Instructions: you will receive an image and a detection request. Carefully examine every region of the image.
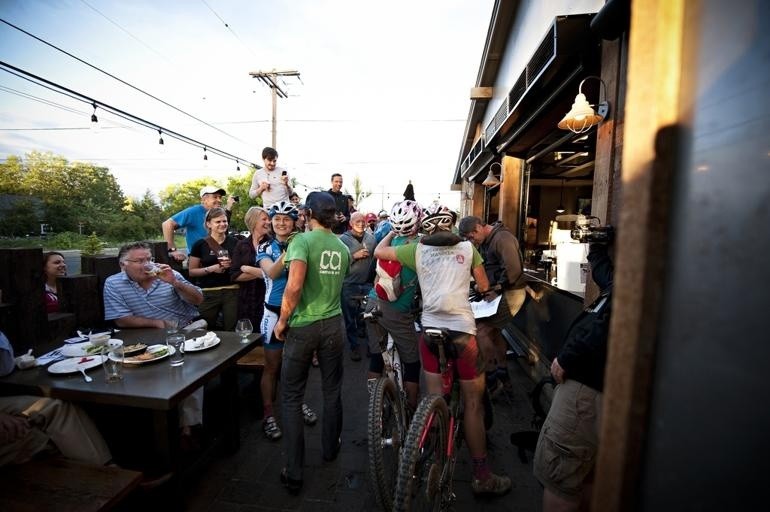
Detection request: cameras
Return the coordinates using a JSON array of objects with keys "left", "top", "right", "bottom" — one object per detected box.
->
[{"left": 233, "top": 196, "right": 240, "bottom": 202}]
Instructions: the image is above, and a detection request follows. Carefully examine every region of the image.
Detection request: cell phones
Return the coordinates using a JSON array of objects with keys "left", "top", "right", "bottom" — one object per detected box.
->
[
  {"left": 64, "top": 336, "right": 89, "bottom": 345},
  {"left": 281, "top": 170, "right": 287, "bottom": 176}
]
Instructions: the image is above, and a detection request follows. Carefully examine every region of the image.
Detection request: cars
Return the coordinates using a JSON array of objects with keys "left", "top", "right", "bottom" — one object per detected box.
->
[{"left": 235, "top": 230, "right": 250, "bottom": 238}]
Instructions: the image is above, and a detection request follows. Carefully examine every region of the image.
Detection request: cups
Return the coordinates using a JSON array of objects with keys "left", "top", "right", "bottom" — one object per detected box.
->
[
  {"left": 141, "top": 261, "right": 167, "bottom": 279},
  {"left": 166, "top": 333, "right": 186, "bottom": 367},
  {"left": 217, "top": 249, "right": 229, "bottom": 267},
  {"left": 101, "top": 344, "right": 124, "bottom": 384}
]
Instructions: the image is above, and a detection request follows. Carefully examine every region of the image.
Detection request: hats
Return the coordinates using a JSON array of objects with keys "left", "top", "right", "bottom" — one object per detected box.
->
[
  {"left": 366, "top": 210, "right": 389, "bottom": 222},
  {"left": 199, "top": 185, "right": 226, "bottom": 198}
]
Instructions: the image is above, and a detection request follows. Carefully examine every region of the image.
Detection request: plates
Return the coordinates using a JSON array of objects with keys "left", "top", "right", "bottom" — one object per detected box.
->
[
  {"left": 183, "top": 336, "right": 222, "bottom": 352},
  {"left": 114, "top": 343, "right": 147, "bottom": 358},
  {"left": 47, "top": 354, "right": 109, "bottom": 374},
  {"left": 62, "top": 338, "right": 123, "bottom": 357},
  {"left": 108, "top": 344, "right": 173, "bottom": 364}
]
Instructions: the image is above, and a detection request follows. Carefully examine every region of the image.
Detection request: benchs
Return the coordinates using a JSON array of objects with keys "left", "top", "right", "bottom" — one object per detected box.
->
[
  {"left": 234, "top": 344, "right": 266, "bottom": 392},
  {"left": 0, "top": 449, "right": 148, "bottom": 512}
]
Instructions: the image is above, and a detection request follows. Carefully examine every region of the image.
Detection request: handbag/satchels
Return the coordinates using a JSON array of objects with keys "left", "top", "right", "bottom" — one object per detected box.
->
[{"left": 375, "top": 258, "right": 403, "bottom": 302}]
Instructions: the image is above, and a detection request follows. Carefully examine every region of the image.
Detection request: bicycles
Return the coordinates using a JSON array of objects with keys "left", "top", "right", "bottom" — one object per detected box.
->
[
  {"left": 352, "top": 294, "right": 424, "bottom": 512},
  {"left": 388, "top": 283, "right": 507, "bottom": 512}
]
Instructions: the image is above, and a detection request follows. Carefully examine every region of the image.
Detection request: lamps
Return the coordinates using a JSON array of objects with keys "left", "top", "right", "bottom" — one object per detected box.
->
[
  {"left": 555, "top": 174, "right": 568, "bottom": 214},
  {"left": 480, "top": 157, "right": 505, "bottom": 192},
  {"left": 556, "top": 73, "right": 611, "bottom": 139}
]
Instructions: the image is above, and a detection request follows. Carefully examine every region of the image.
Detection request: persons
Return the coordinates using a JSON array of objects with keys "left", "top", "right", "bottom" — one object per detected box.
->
[
  {"left": 44, "top": 251, "right": 70, "bottom": 313},
  {"left": 0, "top": 331, "right": 182, "bottom": 490},
  {"left": 101, "top": 146, "right": 617, "bottom": 511}
]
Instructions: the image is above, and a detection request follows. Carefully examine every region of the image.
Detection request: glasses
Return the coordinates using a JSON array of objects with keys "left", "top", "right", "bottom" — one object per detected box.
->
[
  {"left": 467, "top": 230, "right": 476, "bottom": 241},
  {"left": 124, "top": 257, "right": 156, "bottom": 264}
]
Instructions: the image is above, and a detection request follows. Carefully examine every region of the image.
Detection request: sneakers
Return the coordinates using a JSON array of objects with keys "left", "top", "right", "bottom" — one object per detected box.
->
[
  {"left": 472, "top": 472, "right": 511, "bottom": 497},
  {"left": 301, "top": 403, "right": 317, "bottom": 425},
  {"left": 262, "top": 416, "right": 283, "bottom": 440},
  {"left": 351, "top": 346, "right": 362, "bottom": 361}
]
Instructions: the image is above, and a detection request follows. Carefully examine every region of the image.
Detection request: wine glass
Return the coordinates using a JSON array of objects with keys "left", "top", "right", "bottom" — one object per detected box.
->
[{"left": 235, "top": 319, "right": 253, "bottom": 344}]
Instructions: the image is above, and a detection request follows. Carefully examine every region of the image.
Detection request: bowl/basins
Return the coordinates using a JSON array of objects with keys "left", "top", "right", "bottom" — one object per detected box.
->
[{"left": 87, "top": 334, "right": 112, "bottom": 346}]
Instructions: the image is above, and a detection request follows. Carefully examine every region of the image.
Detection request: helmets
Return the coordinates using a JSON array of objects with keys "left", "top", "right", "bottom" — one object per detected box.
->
[
  {"left": 268, "top": 200, "right": 299, "bottom": 221},
  {"left": 421, "top": 204, "right": 453, "bottom": 231},
  {"left": 390, "top": 200, "right": 421, "bottom": 236}
]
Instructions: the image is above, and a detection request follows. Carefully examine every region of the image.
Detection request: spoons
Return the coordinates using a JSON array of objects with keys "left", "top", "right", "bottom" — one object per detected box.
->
[{"left": 76, "top": 365, "right": 93, "bottom": 383}]
